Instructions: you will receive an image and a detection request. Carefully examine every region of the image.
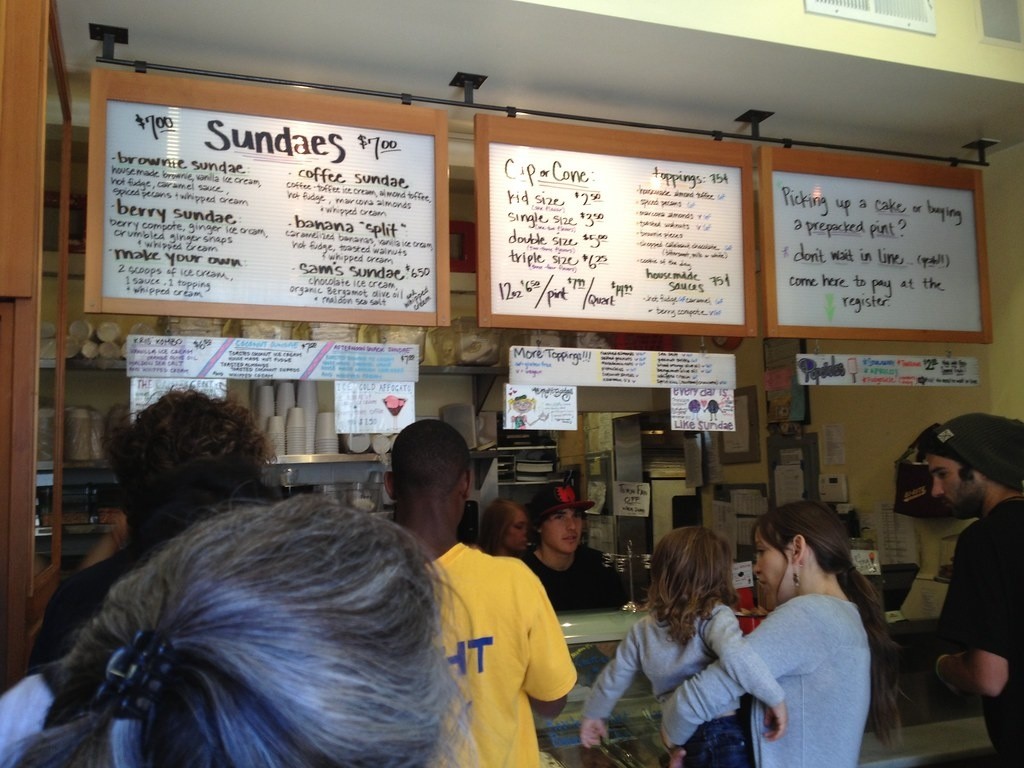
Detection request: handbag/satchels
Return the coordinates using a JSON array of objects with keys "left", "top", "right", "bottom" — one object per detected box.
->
[{"left": 893, "top": 423, "right": 946, "bottom": 519}]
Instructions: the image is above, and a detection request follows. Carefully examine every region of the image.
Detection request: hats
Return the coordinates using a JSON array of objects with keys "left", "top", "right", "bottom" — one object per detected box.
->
[
  {"left": 527, "top": 483, "right": 596, "bottom": 516},
  {"left": 934, "top": 412, "right": 1024, "bottom": 491}
]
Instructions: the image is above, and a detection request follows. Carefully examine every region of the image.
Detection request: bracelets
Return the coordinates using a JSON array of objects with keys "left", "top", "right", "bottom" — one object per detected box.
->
[{"left": 936, "top": 655, "right": 952, "bottom": 679}]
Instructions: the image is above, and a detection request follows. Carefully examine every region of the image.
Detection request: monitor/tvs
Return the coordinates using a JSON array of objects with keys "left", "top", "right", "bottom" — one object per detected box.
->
[{"left": 864, "top": 562, "right": 918, "bottom": 610}]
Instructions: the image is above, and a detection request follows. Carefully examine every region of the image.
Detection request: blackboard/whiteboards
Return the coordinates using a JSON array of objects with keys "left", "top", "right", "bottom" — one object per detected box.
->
[
  {"left": 472, "top": 114, "right": 761, "bottom": 336},
  {"left": 85, "top": 69, "right": 452, "bottom": 326},
  {"left": 757, "top": 143, "right": 995, "bottom": 346}
]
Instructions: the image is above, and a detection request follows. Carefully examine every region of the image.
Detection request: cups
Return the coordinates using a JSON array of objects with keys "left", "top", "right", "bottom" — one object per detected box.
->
[
  {"left": 41, "top": 320, "right": 155, "bottom": 360},
  {"left": 251, "top": 381, "right": 397, "bottom": 456}
]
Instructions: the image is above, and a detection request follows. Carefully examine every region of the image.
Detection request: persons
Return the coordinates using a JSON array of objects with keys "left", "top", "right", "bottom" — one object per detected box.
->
[
  {"left": 0, "top": 388, "right": 465, "bottom": 768},
  {"left": 579, "top": 525, "right": 789, "bottom": 768},
  {"left": 384, "top": 418, "right": 577, "bottom": 768},
  {"left": 660, "top": 500, "right": 903, "bottom": 768},
  {"left": 521, "top": 482, "right": 629, "bottom": 682},
  {"left": 479, "top": 497, "right": 529, "bottom": 558},
  {"left": 927, "top": 412, "right": 1024, "bottom": 768}
]
